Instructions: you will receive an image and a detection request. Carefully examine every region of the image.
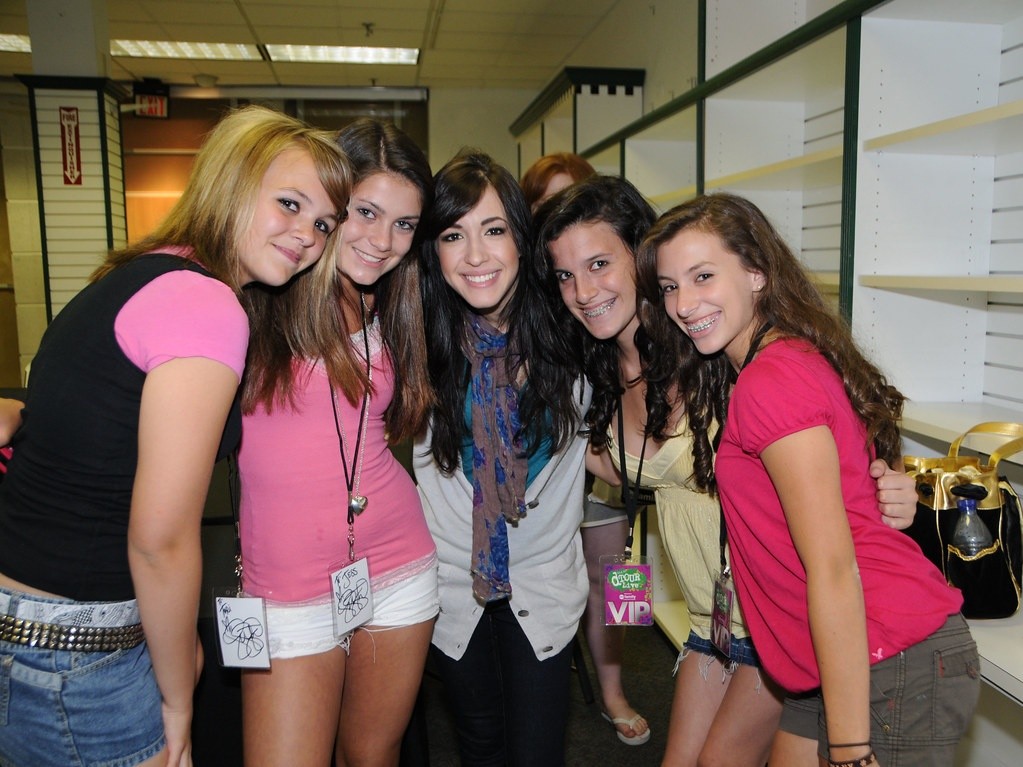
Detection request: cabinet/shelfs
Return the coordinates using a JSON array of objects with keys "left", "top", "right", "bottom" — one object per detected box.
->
[{"left": 508, "top": 0, "right": 1022, "bottom": 708}]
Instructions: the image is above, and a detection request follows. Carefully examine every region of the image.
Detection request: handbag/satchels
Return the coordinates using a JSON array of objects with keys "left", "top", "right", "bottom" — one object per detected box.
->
[{"left": 903, "top": 422, "right": 1023, "bottom": 620}]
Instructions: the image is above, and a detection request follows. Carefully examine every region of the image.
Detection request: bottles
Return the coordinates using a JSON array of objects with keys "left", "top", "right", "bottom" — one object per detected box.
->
[{"left": 954, "top": 499, "right": 993, "bottom": 556}]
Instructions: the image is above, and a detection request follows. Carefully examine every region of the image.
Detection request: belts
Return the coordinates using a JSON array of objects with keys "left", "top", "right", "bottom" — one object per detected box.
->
[{"left": 0, "top": 615, "right": 145, "bottom": 652}]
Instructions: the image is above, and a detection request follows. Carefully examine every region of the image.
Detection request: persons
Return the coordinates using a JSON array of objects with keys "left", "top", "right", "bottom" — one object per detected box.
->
[
  {"left": 523, "top": 154, "right": 651, "bottom": 745},
  {"left": 527, "top": 176, "right": 784, "bottom": 766},
  {"left": 0, "top": 118, "right": 440, "bottom": 766},
  {"left": 634, "top": 194, "right": 980, "bottom": 767},
  {"left": 413, "top": 156, "right": 595, "bottom": 766},
  {"left": 0, "top": 104, "right": 352, "bottom": 766}
]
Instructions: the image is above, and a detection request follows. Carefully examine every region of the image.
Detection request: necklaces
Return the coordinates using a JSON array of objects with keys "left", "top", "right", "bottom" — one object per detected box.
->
[
  {"left": 333, "top": 290, "right": 373, "bottom": 516},
  {"left": 626, "top": 376, "right": 643, "bottom": 388}
]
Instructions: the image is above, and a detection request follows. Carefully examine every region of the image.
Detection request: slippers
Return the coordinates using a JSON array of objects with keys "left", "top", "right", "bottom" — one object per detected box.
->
[{"left": 601, "top": 708, "right": 650, "bottom": 745}]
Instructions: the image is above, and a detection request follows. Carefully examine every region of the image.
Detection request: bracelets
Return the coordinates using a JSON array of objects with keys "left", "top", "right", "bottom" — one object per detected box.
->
[
  {"left": 827, "top": 742, "right": 871, "bottom": 748},
  {"left": 828, "top": 751, "right": 875, "bottom": 767}
]
